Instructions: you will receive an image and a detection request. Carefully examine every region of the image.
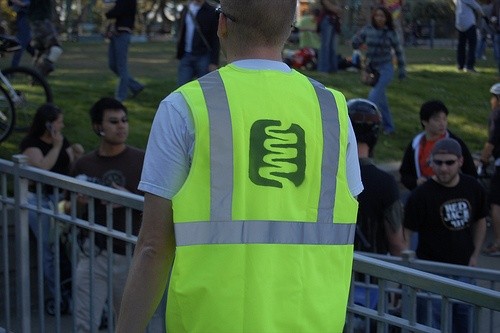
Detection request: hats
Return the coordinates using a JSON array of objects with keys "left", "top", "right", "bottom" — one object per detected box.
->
[{"left": 432, "top": 138, "right": 462, "bottom": 158}]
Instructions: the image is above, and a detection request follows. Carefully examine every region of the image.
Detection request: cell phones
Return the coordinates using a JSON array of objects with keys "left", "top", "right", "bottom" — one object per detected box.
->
[{"left": 46, "top": 122, "right": 56, "bottom": 137}]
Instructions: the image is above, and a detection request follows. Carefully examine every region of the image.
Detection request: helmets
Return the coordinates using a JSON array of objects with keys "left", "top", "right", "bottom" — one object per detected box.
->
[
  {"left": 489, "top": 83, "right": 500, "bottom": 95},
  {"left": 347, "top": 98, "right": 382, "bottom": 138}
]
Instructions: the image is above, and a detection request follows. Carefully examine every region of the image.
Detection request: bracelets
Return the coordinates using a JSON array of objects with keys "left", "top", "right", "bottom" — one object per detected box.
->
[{"left": 478, "top": 159, "right": 487, "bottom": 165}]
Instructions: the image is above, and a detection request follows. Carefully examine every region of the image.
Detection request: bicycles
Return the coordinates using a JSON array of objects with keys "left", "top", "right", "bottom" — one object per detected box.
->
[{"left": 0, "top": 33, "right": 55, "bottom": 144}]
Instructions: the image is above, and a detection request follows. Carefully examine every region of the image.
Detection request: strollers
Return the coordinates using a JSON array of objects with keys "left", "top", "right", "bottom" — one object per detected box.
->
[{"left": 24, "top": 193, "right": 110, "bottom": 331}]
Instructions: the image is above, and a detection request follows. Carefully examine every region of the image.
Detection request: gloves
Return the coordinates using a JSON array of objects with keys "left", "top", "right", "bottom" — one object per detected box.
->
[
  {"left": 398, "top": 69, "right": 405, "bottom": 78},
  {"left": 352, "top": 39, "right": 360, "bottom": 49}
]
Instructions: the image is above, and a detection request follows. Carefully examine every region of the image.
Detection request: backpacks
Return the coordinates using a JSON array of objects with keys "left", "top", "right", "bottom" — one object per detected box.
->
[{"left": 285, "top": 47, "right": 353, "bottom": 71}]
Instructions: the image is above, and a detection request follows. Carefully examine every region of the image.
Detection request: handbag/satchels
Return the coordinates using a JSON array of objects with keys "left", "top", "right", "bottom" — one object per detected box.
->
[
  {"left": 360, "top": 65, "right": 381, "bottom": 86},
  {"left": 335, "top": 15, "right": 342, "bottom": 33},
  {"left": 474, "top": 10, "right": 482, "bottom": 24}
]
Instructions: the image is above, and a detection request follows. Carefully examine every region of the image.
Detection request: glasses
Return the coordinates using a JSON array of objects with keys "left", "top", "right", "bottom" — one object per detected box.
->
[
  {"left": 215, "top": 7, "right": 237, "bottom": 23},
  {"left": 101, "top": 118, "right": 128, "bottom": 125},
  {"left": 433, "top": 160, "right": 457, "bottom": 166}
]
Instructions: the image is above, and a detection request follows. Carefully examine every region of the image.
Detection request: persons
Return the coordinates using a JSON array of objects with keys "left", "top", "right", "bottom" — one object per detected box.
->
[
  {"left": 177, "top": 0, "right": 219, "bottom": 90},
  {"left": 315, "top": 0, "right": 344, "bottom": 75},
  {"left": 402, "top": 137, "right": 488, "bottom": 333},
  {"left": 29, "top": 0, "right": 63, "bottom": 85},
  {"left": 455, "top": 0, "right": 486, "bottom": 74},
  {"left": 352, "top": 6, "right": 407, "bottom": 135},
  {"left": 400, "top": 98, "right": 479, "bottom": 254},
  {"left": 6, "top": 0, "right": 37, "bottom": 79},
  {"left": 342, "top": 97, "right": 405, "bottom": 333},
  {"left": 474, "top": 83, "right": 500, "bottom": 256},
  {"left": 19, "top": 103, "right": 84, "bottom": 317},
  {"left": 72, "top": 95, "right": 146, "bottom": 333},
  {"left": 377, "top": 0, "right": 404, "bottom": 64},
  {"left": 103, "top": 0, "right": 144, "bottom": 102},
  {"left": 475, "top": 0, "right": 500, "bottom": 64},
  {"left": 113, "top": 0, "right": 365, "bottom": 333}
]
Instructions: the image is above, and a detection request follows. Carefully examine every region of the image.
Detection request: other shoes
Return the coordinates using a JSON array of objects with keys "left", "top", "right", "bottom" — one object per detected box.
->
[
  {"left": 457, "top": 52, "right": 500, "bottom": 79},
  {"left": 134, "top": 88, "right": 151, "bottom": 99}
]
